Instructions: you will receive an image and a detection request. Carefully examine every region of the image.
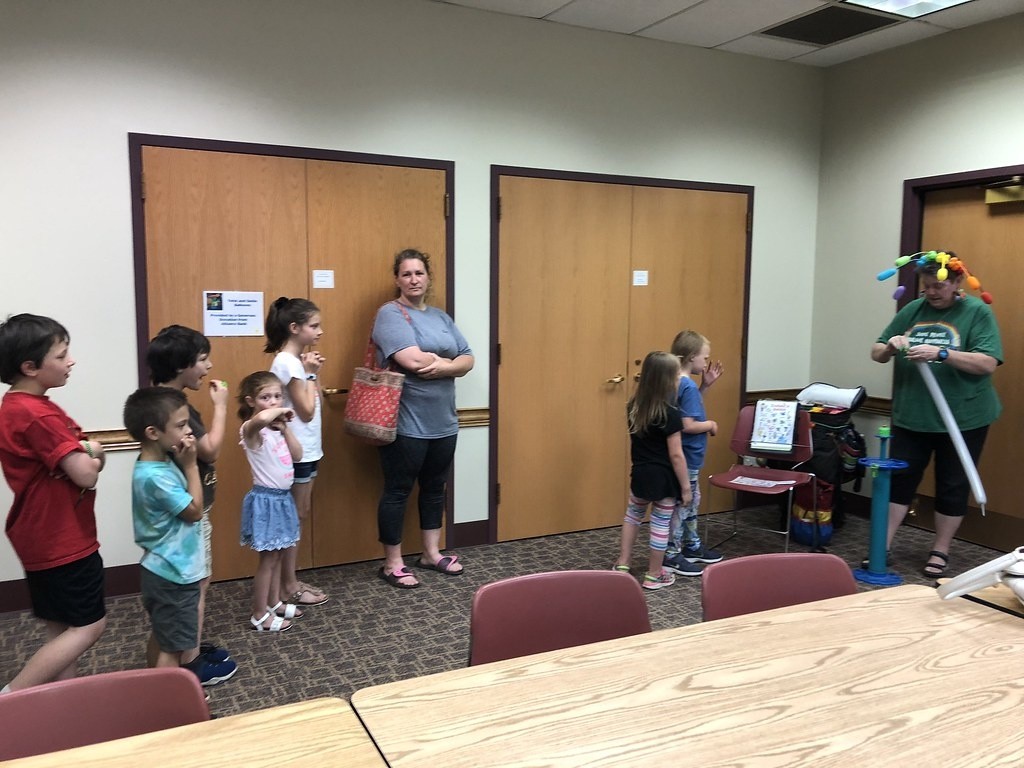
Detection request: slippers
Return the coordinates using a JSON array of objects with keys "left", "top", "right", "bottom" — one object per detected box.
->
[
  {"left": 378, "top": 565, "right": 419, "bottom": 588},
  {"left": 417, "top": 554, "right": 464, "bottom": 575}
]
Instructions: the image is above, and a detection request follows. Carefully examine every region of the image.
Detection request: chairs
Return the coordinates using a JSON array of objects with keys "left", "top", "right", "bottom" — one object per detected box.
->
[
  {"left": 701, "top": 552, "right": 856, "bottom": 622},
  {"left": 0, "top": 666, "right": 210, "bottom": 762},
  {"left": 705, "top": 406, "right": 819, "bottom": 552},
  {"left": 469, "top": 570, "right": 651, "bottom": 668}
]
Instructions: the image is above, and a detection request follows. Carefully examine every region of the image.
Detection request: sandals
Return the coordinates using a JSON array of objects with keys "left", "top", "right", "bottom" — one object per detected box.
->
[
  {"left": 281, "top": 580, "right": 329, "bottom": 606},
  {"left": 859, "top": 549, "right": 893, "bottom": 569},
  {"left": 249, "top": 600, "right": 305, "bottom": 631},
  {"left": 924, "top": 552, "right": 949, "bottom": 578}
]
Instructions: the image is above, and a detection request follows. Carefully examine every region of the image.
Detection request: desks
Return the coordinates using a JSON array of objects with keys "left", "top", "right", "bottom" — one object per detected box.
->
[
  {"left": 352, "top": 584, "right": 1023, "bottom": 768},
  {"left": 938, "top": 577, "right": 1024, "bottom": 619},
  {"left": 0, "top": 697, "right": 389, "bottom": 768}
]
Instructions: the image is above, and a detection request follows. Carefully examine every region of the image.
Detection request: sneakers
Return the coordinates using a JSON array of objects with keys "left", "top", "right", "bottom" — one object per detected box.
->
[
  {"left": 642, "top": 569, "right": 675, "bottom": 590},
  {"left": 612, "top": 558, "right": 631, "bottom": 572},
  {"left": 179, "top": 645, "right": 239, "bottom": 686},
  {"left": 684, "top": 543, "right": 723, "bottom": 564},
  {"left": 662, "top": 553, "right": 703, "bottom": 576}
]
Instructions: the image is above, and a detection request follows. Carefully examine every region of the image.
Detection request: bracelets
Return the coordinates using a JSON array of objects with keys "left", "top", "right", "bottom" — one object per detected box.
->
[{"left": 83, "top": 440, "right": 93, "bottom": 457}]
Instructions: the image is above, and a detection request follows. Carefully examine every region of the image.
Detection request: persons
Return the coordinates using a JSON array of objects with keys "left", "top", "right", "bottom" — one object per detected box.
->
[
  {"left": 264, "top": 296, "right": 329, "bottom": 607},
  {"left": 144, "top": 325, "right": 238, "bottom": 687},
  {"left": 611, "top": 350, "right": 693, "bottom": 589},
  {"left": 859, "top": 250, "right": 1005, "bottom": 578},
  {"left": 0, "top": 313, "right": 106, "bottom": 695},
  {"left": 123, "top": 387, "right": 210, "bottom": 702},
  {"left": 236, "top": 370, "right": 305, "bottom": 632},
  {"left": 372, "top": 249, "right": 475, "bottom": 589},
  {"left": 661, "top": 328, "right": 724, "bottom": 576}
]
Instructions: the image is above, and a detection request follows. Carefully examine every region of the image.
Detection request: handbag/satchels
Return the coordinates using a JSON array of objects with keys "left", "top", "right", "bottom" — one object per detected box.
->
[{"left": 342, "top": 301, "right": 411, "bottom": 446}]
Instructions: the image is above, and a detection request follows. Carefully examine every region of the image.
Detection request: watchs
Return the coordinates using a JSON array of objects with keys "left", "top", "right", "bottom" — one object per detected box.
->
[
  {"left": 937, "top": 346, "right": 949, "bottom": 362},
  {"left": 306, "top": 374, "right": 316, "bottom": 381}
]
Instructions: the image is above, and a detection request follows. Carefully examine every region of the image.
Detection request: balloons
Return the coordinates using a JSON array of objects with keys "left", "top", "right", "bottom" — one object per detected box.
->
[
  {"left": 913, "top": 360, "right": 987, "bottom": 516},
  {"left": 877, "top": 251, "right": 994, "bottom": 304}
]
